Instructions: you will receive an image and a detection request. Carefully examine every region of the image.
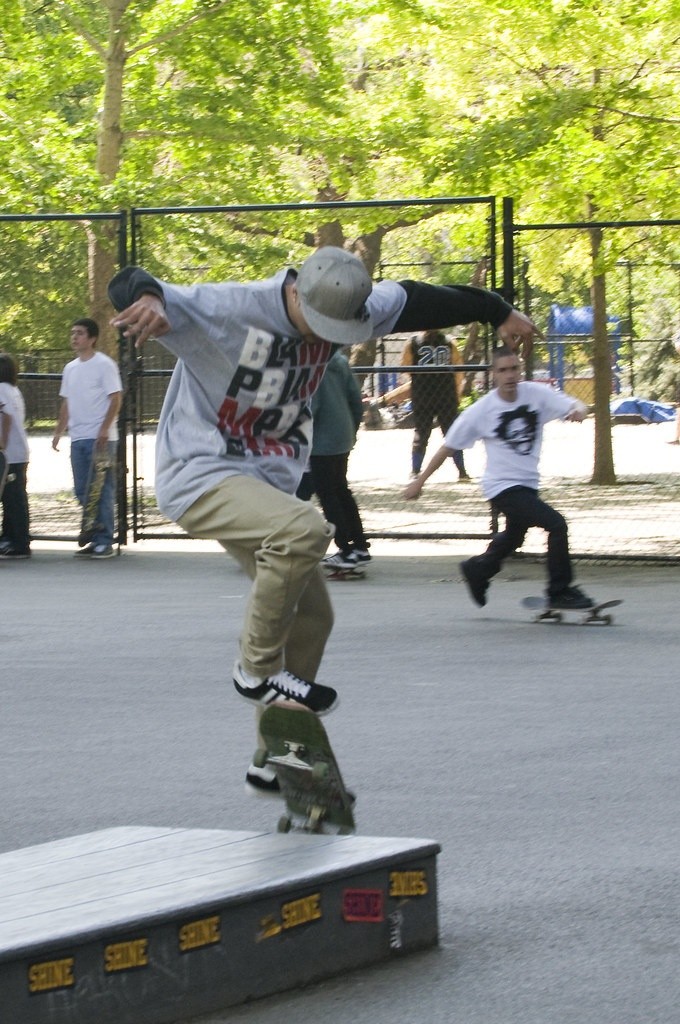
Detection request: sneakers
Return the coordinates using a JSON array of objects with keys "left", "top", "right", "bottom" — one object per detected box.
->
[
  {"left": 90, "top": 545, "right": 113, "bottom": 559},
  {"left": 0, "top": 544, "right": 31, "bottom": 558},
  {"left": 233, "top": 658, "right": 338, "bottom": 717},
  {"left": 73, "top": 544, "right": 95, "bottom": 556},
  {"left": 319, "top": 549, "right": 373, "bottom": 568},
  {"left": 549, "top": 582, "right": 595, "bottom": 608},
  {"left": 244, "top": 763, "right": 358, "bottom": 812},
  {"left": 458, "top": 558, "right": 490, "bottom": 608}
]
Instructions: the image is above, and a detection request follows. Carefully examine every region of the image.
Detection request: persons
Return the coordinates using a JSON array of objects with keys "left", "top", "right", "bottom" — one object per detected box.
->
[
  {"left": 107, "top": 246, "right": 543, "bottom": 805},
  {"left": 53, "top": 319, "right": 122, "bottom": 558},
  {"left": 400, "top": 346, "right": 593, "bottom": 610},
  {"left": 394, "top": 327, "right": 473, "bottom": 484},
  {"left": 293, "top": 352, "right": 372, "bottom": 568},
  {"left": 0, "top": 352, "right": 32, "bottom": 560}
]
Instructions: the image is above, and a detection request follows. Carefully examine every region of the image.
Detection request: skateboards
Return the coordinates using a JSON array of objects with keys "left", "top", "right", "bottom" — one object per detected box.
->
[
  {"left": 76, "top": 438, "right": 112, "bottom": 547},
  {"left": 259, "top": 700, "right": 358, "bottom": 837},
  {"left": 0, "top": 448, "right": 16, "bottom": 501},
  {"left": 321, "top": 563, "right": 370, "bottom": 582},
  {"left": 521, "top": 597, "right": 625, "bottom": 626}
]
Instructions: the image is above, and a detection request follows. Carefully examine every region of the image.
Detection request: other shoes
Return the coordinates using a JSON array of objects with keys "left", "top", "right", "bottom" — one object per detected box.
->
[{"left": 459, "top": 471, "right": 472, "bottom": 480}]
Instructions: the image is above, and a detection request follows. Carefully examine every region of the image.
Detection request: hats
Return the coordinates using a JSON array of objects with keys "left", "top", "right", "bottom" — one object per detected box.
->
[{"left": 296, "top": 246, "right": 373, "bottom": 344}]
